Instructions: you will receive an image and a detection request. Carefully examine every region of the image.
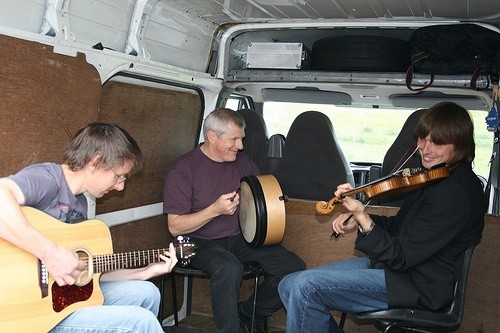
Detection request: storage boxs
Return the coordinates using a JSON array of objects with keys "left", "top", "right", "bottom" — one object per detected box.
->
[{"left": 246, "top": 41, "right": 310, "bottom": 71}]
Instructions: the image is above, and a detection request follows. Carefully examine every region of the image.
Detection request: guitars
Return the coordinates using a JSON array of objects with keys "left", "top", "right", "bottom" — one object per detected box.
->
[{"left": 0, "top": 205, "right": 198, "bottom": 333}]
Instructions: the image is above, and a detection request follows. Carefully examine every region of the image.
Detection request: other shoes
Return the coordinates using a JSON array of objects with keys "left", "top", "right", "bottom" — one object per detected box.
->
[{"left": 236, "top": 302, "right": 274, "bottom": 333}]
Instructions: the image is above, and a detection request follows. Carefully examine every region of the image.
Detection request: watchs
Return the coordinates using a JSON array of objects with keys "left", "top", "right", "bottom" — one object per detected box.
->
[{"left": 358, "top": 220, "right": 375, "bottom": 236}]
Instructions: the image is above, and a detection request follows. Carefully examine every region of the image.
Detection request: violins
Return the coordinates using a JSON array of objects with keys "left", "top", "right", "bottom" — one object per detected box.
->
[{"left": 315, "top": 162, "right": 452, "bottom": 216}]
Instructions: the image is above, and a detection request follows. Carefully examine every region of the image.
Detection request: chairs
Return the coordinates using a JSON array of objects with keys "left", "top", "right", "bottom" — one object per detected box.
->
[
  {"left": 159, "top": 260, "right": 266, "bottom": 333},
  {"left": 340, "top": 238, "right": 482, "bottom": 333},
  {"left": 238, "top": 108, "right": 429, "bottom": 206}
]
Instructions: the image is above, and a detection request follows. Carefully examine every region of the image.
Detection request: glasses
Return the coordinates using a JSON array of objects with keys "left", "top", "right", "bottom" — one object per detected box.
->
[{"left": 101, "top": 160, "right": 128, "bottom": 183}]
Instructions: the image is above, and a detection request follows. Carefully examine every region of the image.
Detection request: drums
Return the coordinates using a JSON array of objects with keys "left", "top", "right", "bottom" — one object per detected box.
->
[{"left": 237, "top": 174, "right": 286, "bottom": 247}]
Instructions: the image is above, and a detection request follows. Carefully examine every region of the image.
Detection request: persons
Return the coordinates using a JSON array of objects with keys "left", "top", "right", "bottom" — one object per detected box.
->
[
  {"left": 0, "top": 123, "right": 178, "bottom": 333},
  {"left": 277, "top": 102, "right": 486, "bottom": 333},
  {"left": 163, "top": 108, "right": 306, "bottom": 333}
]
